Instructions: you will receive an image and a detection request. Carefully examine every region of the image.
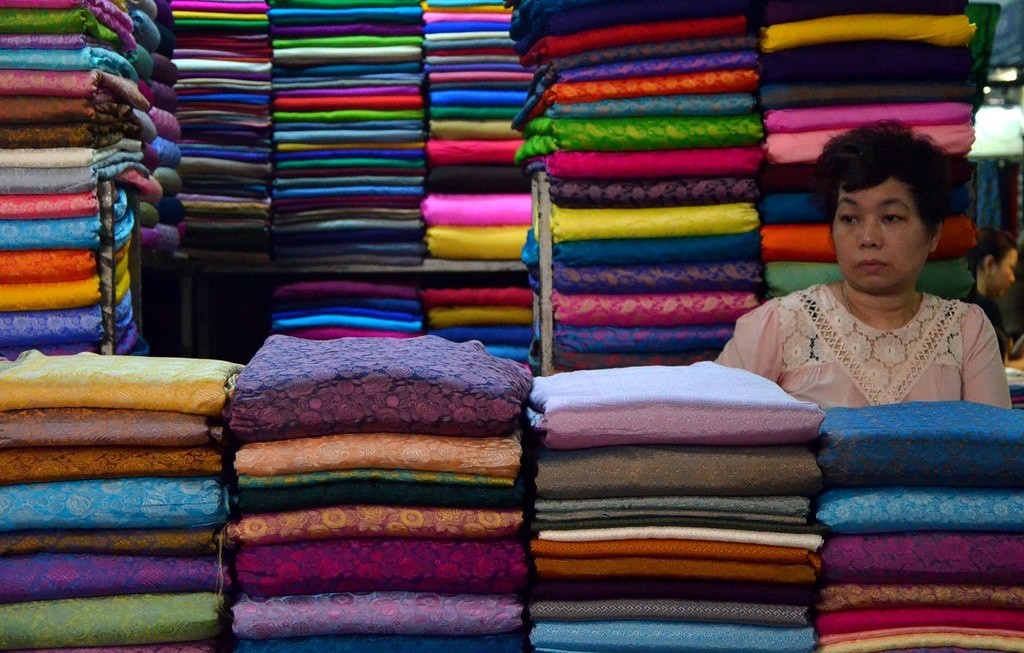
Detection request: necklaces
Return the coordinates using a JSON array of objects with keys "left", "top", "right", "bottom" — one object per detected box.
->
[{"left": 842, "top": 287, "right": 915, "bottom": 317}]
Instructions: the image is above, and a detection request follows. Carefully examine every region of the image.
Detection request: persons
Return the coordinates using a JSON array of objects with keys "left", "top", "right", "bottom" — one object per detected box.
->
[
  {"left": 715, "top": 123, "right": 1011, "bottom": 413},
  {"left": 960, "top": 226, "right": 1018, "bottom": 364}
]
